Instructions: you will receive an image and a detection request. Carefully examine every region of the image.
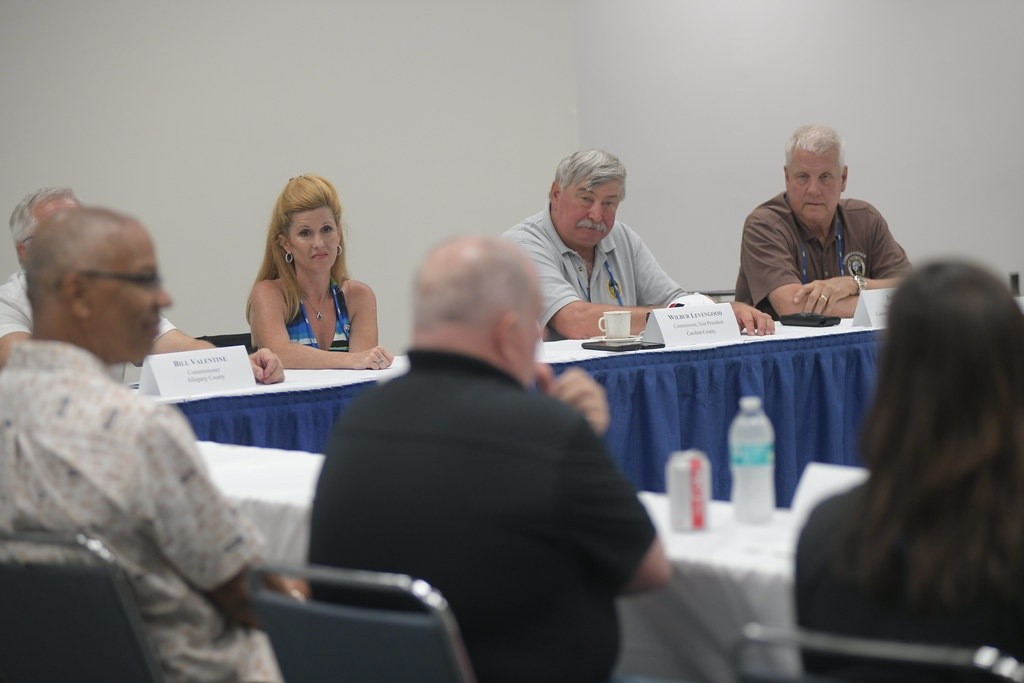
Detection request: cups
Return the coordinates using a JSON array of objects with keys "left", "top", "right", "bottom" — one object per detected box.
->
[{"left": 598, "top": 312, "right": 631, "bottom": 337}]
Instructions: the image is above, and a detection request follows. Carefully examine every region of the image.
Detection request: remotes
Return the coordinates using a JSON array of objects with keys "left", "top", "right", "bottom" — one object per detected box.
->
[{"left": 780, "top": 311, "right": 841, "bottom": 326}]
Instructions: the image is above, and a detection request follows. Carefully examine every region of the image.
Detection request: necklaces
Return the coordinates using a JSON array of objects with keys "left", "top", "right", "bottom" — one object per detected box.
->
[{"left": 303, "top": 292, "right": 330, "bottom": 322}]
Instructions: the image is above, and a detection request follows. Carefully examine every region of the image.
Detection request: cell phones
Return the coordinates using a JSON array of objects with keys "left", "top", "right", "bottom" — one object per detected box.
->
[{"left": 582, "top": 340, "right": 642, "bottom": 352}]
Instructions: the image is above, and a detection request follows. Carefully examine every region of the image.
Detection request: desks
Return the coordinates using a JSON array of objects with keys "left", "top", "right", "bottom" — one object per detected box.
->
[
  {"left": 156, "top": 318, "right": 889, "bottom": 513},
  {"left": 201, "top": 439, "right": 804, "bottom": 683}
]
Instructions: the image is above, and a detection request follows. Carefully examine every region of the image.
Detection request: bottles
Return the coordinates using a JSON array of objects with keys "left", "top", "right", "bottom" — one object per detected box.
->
[{"left": 730, "top": 396, "right": 773, "bottom": 521}]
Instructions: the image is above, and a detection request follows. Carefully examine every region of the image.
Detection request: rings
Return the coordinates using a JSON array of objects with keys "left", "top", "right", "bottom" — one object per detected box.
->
[
  {"left": 378, "top": 359, "right": 384, "bottom": 364},
  {"left": 821, "top": 295, "right": 828, "bottom": 301}
]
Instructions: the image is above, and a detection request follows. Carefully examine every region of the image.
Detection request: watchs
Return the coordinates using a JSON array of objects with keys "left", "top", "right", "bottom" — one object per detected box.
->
[{"left": 853, "top": 275, "right": 867, "bottom": 295}]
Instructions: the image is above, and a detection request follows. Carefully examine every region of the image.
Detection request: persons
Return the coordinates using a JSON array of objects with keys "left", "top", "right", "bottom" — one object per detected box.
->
[
  {"left": 0, "top": 187, "right": 285, "bottom": 385},
  {"left": 243, "top": 176, "right": 394, "bottom": 372},
  {"left": 499, "top": 149, "right": 775, "bottom": 336},
  {"left": 789, "top": 260, "right": 1024, "bottom": 683},
  {"left": 305, "top": 232, "right": 673, "bottom": 683},
  {"left": 0, "top": 207, "right": 308, "bottom": 683},
  {"left": 734, "top": 124, "right": 912, "bottom": 318}
]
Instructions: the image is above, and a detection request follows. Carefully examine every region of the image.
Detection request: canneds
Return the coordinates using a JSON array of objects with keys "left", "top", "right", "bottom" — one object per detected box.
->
[{"left": 665, "top": 448, "right": 713, "bottom": 532}]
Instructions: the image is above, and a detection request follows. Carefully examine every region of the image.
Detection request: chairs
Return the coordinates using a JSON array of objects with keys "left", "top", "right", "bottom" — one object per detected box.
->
[
  {"left": 0, "top": 528, "right": 166, "bottom": 683},
  {"left": 244, "top": 556, "right": 478, "bottom": 683},
  {"left": 730, "top": 621, "right": 1024, "bottom": 683}
]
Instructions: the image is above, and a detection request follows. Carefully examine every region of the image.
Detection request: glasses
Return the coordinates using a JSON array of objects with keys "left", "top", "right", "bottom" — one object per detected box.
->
[{"left": 80, "top": 265, "right": 159, "bottom": 290}]
